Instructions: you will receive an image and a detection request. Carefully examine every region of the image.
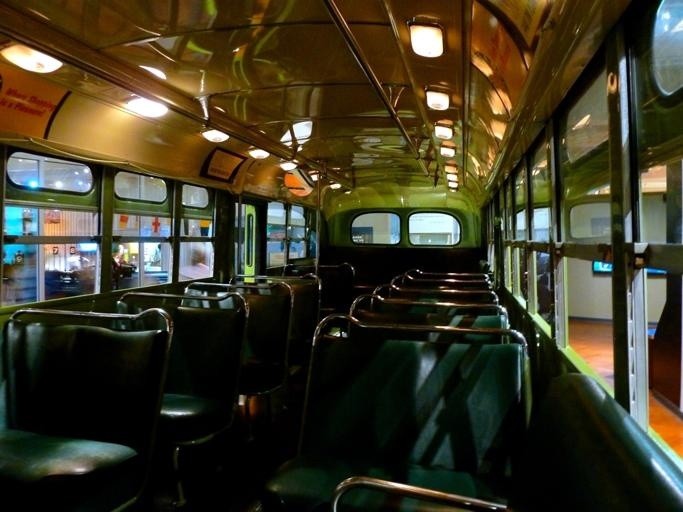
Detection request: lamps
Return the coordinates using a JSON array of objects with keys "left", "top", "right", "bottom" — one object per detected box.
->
[
  {"left": 424, "top": 85, "right": 451, "bottom": 112},
  {"left": 434, "top": 121, "right": 453, "bottom": 140},
  {"left": 120, "top": 95, "right": 168, "bottom": 118},
  {"left": 407, "top": 18, "right": 448, "bottom": 58},
  {"left": 444, "top": 165, "right": 458, "bottom": 192},
  {"left": 279, "top": 157, "right": 352, "bottom": 195},
  {"left": 201, "top": 129, "right": 229, "bottom": 143},
  {"left": 249, "top": 146, "right": 271, "bottom": 158},
  {"left": 440, "top": 145, "right": 455, "bottom": 158},
  {"left": 0, "top": 40, "right": 63, "bottom": 74}
]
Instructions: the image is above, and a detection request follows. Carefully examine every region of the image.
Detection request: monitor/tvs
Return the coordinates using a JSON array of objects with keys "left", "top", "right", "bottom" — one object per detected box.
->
[{"left": 592, "top": 261, "right": 667, "bottom": 275}]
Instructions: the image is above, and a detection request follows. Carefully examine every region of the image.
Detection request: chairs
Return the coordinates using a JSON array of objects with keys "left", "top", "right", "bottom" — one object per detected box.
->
[
  {"left": 254, "top": 262, "right": 682, "bottom": 512},
  {"left": 1, "top": 260, "right": 352, "bottom": 512}
]
